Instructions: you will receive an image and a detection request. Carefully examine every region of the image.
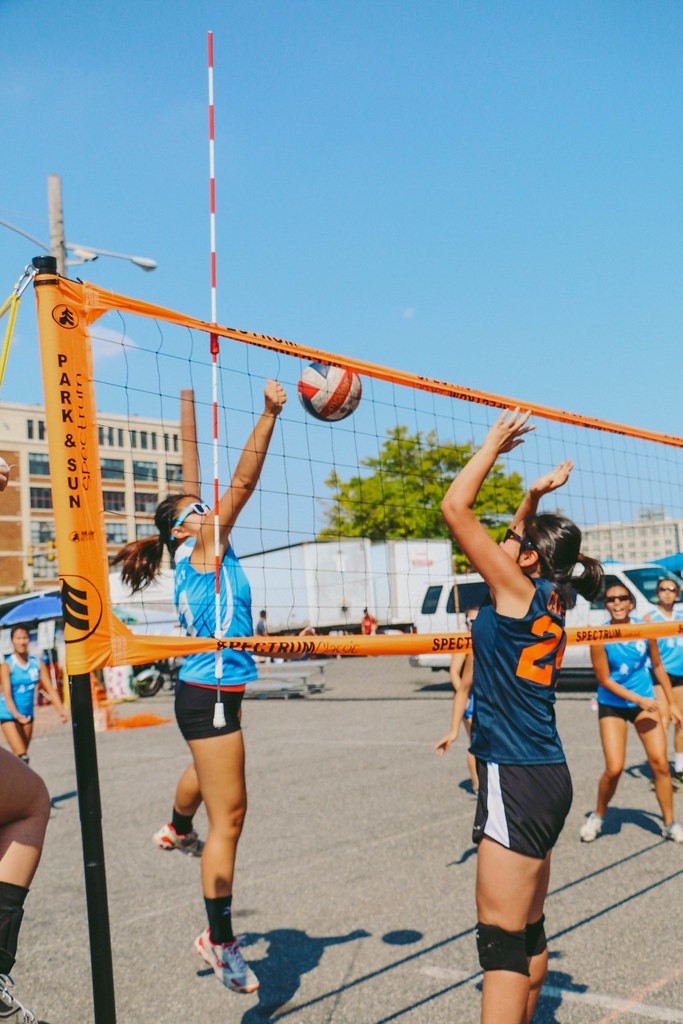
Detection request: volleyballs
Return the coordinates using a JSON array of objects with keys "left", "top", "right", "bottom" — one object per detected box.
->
[{"left": 297, "top": 359, "right": 362, "bottom": 423}]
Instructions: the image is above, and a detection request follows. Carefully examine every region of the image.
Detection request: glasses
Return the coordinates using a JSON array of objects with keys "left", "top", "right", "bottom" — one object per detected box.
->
[
  {"left": 503, "top": 529, "right": 537, "bottom": 551},
  {"left": 657, "top": 586, "right": 678, "bottom": 593},
  {"left": 170, "top": 499, "right": 212, "bottom": 542},
  {"left": 603, "top": 594, "right": 630, "bottom": 603},
  {"left": 466, "top": 618, "right": 474, "bottom": 625}
]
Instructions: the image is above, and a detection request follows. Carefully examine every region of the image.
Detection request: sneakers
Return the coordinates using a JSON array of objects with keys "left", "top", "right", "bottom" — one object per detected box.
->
[
  {"left": 153, "top": 824, "right": 206, "bottom": 858},
  {"left": 581, "top": 815, "right": 602, "bottom": 842},
  {"left": 661, "top": 822, "right": 683, "bottom": 843},
  {"left": 0, "top": 974, "right": 35, "bottom": 1024},
  {"left": 195, "top": 925, "right": 261, "bottom": 993}
]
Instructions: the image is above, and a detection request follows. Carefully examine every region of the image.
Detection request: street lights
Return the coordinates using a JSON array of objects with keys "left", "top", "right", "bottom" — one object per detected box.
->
[{"left": 46, "top": 172, "right": 160, "bottom": 286}]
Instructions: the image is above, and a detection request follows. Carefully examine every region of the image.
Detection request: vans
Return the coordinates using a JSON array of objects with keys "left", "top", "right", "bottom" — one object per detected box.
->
[{"left": 407, "top": 559, "right": 683, "bottom": 695}]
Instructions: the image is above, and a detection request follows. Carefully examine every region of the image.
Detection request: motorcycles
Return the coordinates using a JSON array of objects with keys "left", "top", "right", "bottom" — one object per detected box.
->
[{"left": 133, "top": 656, "right": 187, "bottom": 698}]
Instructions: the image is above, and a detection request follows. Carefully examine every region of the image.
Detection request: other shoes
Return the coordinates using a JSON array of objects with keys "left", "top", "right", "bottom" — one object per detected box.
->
[
  {"left": 649, "top": 775, "right": 682, "bottom": 793},
  {"left": 675, "top": 769, "right": 683, "bottom": 783}
]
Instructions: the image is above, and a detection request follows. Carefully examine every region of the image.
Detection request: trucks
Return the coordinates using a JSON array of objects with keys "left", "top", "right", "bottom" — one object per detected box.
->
[{"left": 234, "top": 536, "right": 454, "bottom": 664}]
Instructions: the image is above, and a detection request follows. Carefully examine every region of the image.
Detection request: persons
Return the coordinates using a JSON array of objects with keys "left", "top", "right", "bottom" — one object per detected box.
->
[
  {"left": 0, "top": 626, "right": 68, "bottom": 767},
  {"left": 361, "top": 609, "right": 378, "bottom": 635},
  {"left": 434, "top": 605, "right": 479, "bottom": 796},
  {"left": 579, "top": 583, "right": 683, "bottom": 844},
  {"left": 642, "top": 578, "right": 683, "bottom": 796},
  {"left": 111, "top": 376, "right": 288, "bottom": 994},
  {"left": 0, "top": 746, "right": 51, "bottom": 1024},
  {"left": 438, "top": 406, "right": 603, "bottom": 1024},
  {"left": 256, "top": 610, "right": 269, "bottom": 636}
]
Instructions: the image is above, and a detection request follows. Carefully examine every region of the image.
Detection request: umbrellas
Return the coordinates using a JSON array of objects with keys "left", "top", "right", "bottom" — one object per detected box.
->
[{"left": 0, "top": 594, "right": 63, "bottom": 684}]
